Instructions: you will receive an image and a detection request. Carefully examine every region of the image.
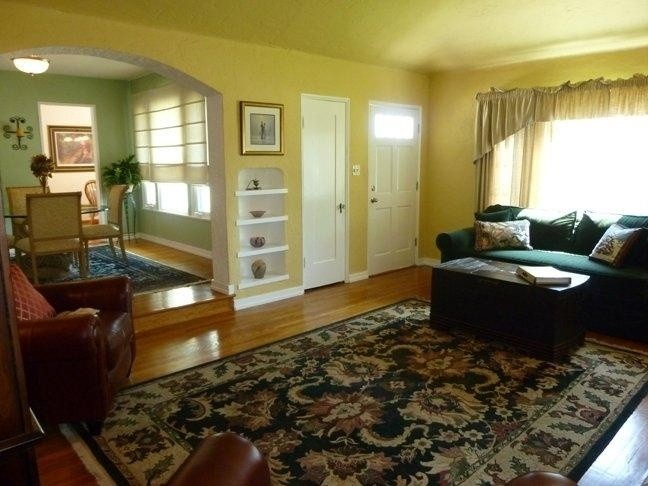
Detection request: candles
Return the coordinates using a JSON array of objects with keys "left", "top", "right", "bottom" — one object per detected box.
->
[{"left": 15, "top": 127, "right": 24, "bottom": 137}]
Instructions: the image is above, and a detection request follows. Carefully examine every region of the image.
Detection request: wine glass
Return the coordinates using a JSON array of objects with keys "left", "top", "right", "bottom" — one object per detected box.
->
[{"left": 252, "top": 180, "right": 260, "bottom": 189}]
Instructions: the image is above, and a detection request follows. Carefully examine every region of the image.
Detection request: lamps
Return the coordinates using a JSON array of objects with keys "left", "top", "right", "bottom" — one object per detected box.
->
[{"left": 9, "top": 54, "right": 54, "bottom": 78}]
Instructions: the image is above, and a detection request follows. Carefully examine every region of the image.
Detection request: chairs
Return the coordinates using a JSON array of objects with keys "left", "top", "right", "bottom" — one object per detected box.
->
[
  {"left": 84, "top": 178, "right": 97, "bottom": 225},
  {"left": 10, "top": 191, "right": 88, "bottom": 286},
  {"left": 72, "top": 182, "right": 130, "bottom": 277},
  {"left": 5, "top": 257, "right": 138, "bottom": 439},
  {"left": 160, "top": 429, "right": 581, "bottom": 485},
  {"left": 5, "top": 184, "right": 51, "bottom": 268}
]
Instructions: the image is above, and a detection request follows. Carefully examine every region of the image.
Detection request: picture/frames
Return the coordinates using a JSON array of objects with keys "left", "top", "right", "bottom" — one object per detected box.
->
[
  {"left": 237, "top": 100, "right": 286, "bottom": 156},
  {"left": 44, "top": 122, "right": 96, "bottom": 175}
]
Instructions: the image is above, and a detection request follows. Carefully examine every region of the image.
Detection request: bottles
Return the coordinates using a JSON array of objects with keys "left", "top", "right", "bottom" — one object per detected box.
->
[{"left": 251, "top": 260, "right": 266, "bottom": 279}]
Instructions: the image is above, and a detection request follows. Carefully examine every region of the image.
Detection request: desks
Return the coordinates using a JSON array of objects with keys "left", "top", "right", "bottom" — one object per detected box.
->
[
  {"left": 1, "top": 203, "right": 112, "bottom": 284},
  {"left": 121, "top": 191, "right": 139, "bottom": 245}
]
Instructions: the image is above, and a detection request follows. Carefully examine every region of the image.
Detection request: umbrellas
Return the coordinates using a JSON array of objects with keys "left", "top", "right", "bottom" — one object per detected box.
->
[{"left": 516, "top": 265, "right": 571, "bottom": 286}]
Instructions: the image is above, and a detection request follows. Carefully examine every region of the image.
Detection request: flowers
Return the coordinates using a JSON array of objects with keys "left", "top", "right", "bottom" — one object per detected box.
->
[{"left": 28, "top": 153, "right": 56, "bottom": 193}]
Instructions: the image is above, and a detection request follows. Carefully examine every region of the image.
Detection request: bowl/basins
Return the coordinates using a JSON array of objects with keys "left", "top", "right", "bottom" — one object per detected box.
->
[
  {"left": 250, "top": 211, "right": 266, "bottom": 217},
  {"left": 250, "top": 236, "right": 265, "bottom": 247}
]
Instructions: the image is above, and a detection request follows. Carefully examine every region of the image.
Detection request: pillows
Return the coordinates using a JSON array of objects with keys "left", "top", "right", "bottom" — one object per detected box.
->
[
  {"left": 8, "top": 261, "right": 57, "bottom": 323},
  {"left": 466, "top": 205, "right": 648, "bottom": 268}
]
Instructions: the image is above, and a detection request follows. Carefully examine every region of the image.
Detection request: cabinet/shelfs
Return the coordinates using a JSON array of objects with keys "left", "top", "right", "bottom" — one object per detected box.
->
[{"left": 231, "top": 185, "right": 291, "bottom": 291}]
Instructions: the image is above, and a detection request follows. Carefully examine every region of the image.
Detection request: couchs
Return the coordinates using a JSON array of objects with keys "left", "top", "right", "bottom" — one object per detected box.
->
[{"left": 427, "top": 198, "right": 648, "bottom": 355}]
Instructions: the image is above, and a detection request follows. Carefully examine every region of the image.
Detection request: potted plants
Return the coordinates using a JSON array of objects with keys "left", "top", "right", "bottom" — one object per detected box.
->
[{"left": 101, "top": 150, "right": 143, "bottom": 195}]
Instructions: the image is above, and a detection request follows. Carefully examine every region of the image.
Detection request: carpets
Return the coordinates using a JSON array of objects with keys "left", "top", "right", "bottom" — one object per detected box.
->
[
  {"left": 5, "top": 245, "right": 210, "bottom": 305},
  {"left": 51, "top": 289, "right": 647, "bottom": 485}
]
